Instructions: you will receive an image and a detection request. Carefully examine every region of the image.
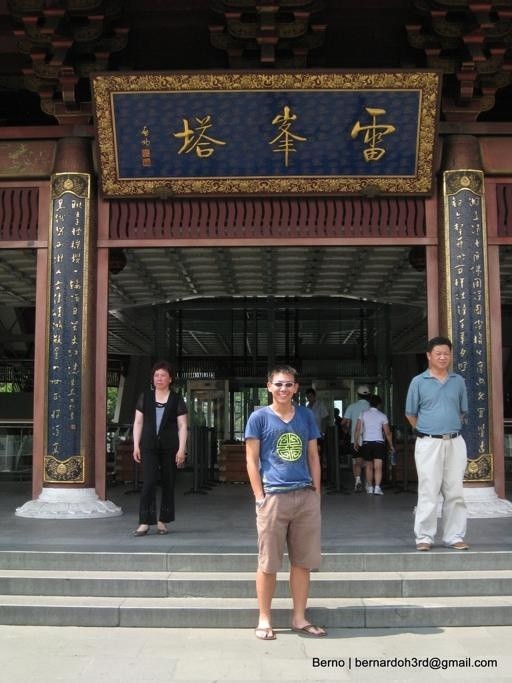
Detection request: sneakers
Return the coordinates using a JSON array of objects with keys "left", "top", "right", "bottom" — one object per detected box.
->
[
  {"left": 354, "top": 476, "right": 385, "bottom": 496},
  {"left": 449, "top": 541, "right": 469, "bottom": 550},
  {"left": 415, "top": 543, "right": 431, "bottom": 551}
]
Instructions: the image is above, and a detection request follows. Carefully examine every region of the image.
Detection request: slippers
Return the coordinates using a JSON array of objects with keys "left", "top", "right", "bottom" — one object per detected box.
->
[
  {"left": 291, "top": 624, "right": 327, "bottom": 637},
  {"left": 255, "top": 626, "right": 276, "bottom": 640}
]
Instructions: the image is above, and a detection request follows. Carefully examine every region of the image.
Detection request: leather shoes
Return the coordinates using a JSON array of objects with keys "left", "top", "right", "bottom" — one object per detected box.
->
[
  {"left": 132, "top": 525, "right": 150, "bottom": 536},
  {"left": 157, "top": 524, "right": 168, "bottom": 535}
]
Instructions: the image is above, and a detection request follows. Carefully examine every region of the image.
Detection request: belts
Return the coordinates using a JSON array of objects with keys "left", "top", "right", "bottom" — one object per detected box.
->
[{"left": 416, "top": 431, "right": 462, "bottom": 440}]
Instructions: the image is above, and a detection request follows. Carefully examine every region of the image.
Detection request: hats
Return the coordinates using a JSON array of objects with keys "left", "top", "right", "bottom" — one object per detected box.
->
[{"left": 357, "top": 386, "right": 371, "bottom": 395}]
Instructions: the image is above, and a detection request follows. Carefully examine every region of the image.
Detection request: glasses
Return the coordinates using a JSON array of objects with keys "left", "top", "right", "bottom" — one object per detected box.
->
[{"left": 270, "top": 381, "right": 294, "bottom": 387}]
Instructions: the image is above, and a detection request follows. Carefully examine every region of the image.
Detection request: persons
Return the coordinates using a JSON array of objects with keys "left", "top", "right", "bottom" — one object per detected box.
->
[
  {"left": 340, "top": 386, "right": 368, "bottom": 493},
  {"left": 405, "top": 338, "right": 469, "bottom": 550},
  {"left": 132, "top": 361, "right": 188, "bottom": 535},
  {"left": 305, "top": 387, "right": 329, "bottom": 483},
  {"left": 244, "top": 364, "right": 327, "bottom": 639},
  {"left": 354, "top": 395, "right": 396, "bottom": 496},
  {"left": 334, "top": 408, "right": 343, "bottom": 441}
]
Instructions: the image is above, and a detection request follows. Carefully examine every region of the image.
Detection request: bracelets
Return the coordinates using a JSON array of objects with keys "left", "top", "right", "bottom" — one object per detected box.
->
[{"left": 321, "top": 432, "right": 326, "bottom": 436}]
